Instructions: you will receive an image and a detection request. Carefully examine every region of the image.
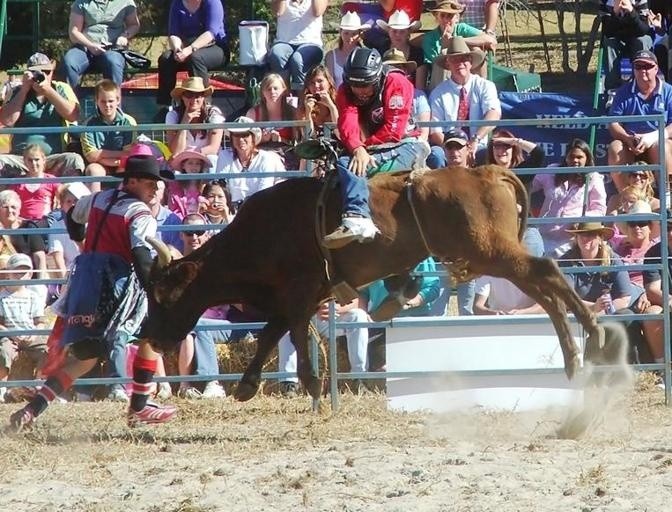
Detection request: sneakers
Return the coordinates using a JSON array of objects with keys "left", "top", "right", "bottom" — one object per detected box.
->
[
  {"left": 128, "top": 401, "right": 180, "bottom": 425},
  {"left": 108, "top": 382, "right": 129, "bottom": 401},
  {"left": 8, "top": 407, "right": 37, "bottom": 431},
  {"left": 178, "top": 387, "right": 205, "bottom": 403},
  {"left": 201, "top": 379, "right": 228, "bottom": 400},
  {"left": 156, "top": 382, "right": 173, "bottom": 401},
  {"left": 318, "top": 216, "right": 375, "bottom": 248},
  {"left": 280, "top": 379, "right": 299, "bottom": 398}
]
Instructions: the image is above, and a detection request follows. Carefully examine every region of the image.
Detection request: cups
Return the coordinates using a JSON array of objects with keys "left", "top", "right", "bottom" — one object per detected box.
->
[{"left": 599, "top": 293, "right": 618, "bottom": 317}]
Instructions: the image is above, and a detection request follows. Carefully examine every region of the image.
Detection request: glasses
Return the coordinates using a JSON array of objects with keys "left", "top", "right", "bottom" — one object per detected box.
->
[
  {"left": 185, "top": 227, "right": 206, "bottom": 237},
  {"left": 632, "top": 64, "right": 653, "bottom": 71}
]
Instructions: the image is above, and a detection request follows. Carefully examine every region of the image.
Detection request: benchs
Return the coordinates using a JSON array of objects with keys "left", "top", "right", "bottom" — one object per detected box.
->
[{"left": 0, "top": 45, "right": 495, "bottom": 109}]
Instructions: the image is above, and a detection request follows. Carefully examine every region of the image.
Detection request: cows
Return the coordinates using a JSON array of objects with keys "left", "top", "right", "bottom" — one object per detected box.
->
[{"left": 144, "top": 164, "right": 605, "bottom": 413}]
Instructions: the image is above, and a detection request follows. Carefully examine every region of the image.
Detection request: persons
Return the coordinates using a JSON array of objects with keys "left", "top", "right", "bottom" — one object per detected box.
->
[
  {"left": 0, "top": 0, "right": 672, "bottom": 403},
  {"left": 11, "top": 154, "right": 181, "bottom": 435}
]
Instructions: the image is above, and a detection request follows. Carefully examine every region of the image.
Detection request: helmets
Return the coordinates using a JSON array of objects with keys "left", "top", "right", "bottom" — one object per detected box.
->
[{"left": 341, "top": 46, "right": 385, "bottom": 88}]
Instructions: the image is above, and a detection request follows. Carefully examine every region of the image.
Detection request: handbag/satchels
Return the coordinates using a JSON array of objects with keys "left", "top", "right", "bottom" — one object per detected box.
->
[{"left": 119, "top": 46, "right": 153, "bottom": 70}]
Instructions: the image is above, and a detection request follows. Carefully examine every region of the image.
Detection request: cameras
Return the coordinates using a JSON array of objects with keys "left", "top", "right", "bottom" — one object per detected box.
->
[
  {"left": 206, "top": 201, "right": 223, "bottom": 212},
  {"left": 603, "top": 295, "right": 616, "bottom": 314},
  {"left": 307, "top": 92, "right": 326, "bottom": 102},
  {"left": 32, "top": 70, "right": 45, "bottom": 83}
]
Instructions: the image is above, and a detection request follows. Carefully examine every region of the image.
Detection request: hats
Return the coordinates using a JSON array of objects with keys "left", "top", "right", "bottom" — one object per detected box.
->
[
  {"left": 615, "top": 201, "right": 661, "bottom": 239},
  {"left": 631, "top": 51, "right": 659, "bottom": 67},
  {"left": 434, "top": 34, "right": 486, "bottom": 70},
  {"left": 336, "top": 9, "right": 423, "bottom": 33},
  {"left": 28, "top": 52, "right": 54, "bottom": 72},
  {"left": 113, "top": 152, "right": 175, "bottom": 184},
  {"left": 565, "top": 221, "right": 615, "bottom": 242},
  {"left": 440, "top": 126, "right": 470, "bottom": 148},
  {"left": 426, "top": 0, "right": 467, "bottom": 16},
  {"left": 218, "top": 114, "right": 263, "bottom": 144},
  {"left": 4, "top": 251, "right": 33, "bottom": 270},
  {"left": 170, "top": 143, "right": 212, "bottom": 170},
  {"left": 170, "top": 76, "right": 213, "bottom": 97}
]
